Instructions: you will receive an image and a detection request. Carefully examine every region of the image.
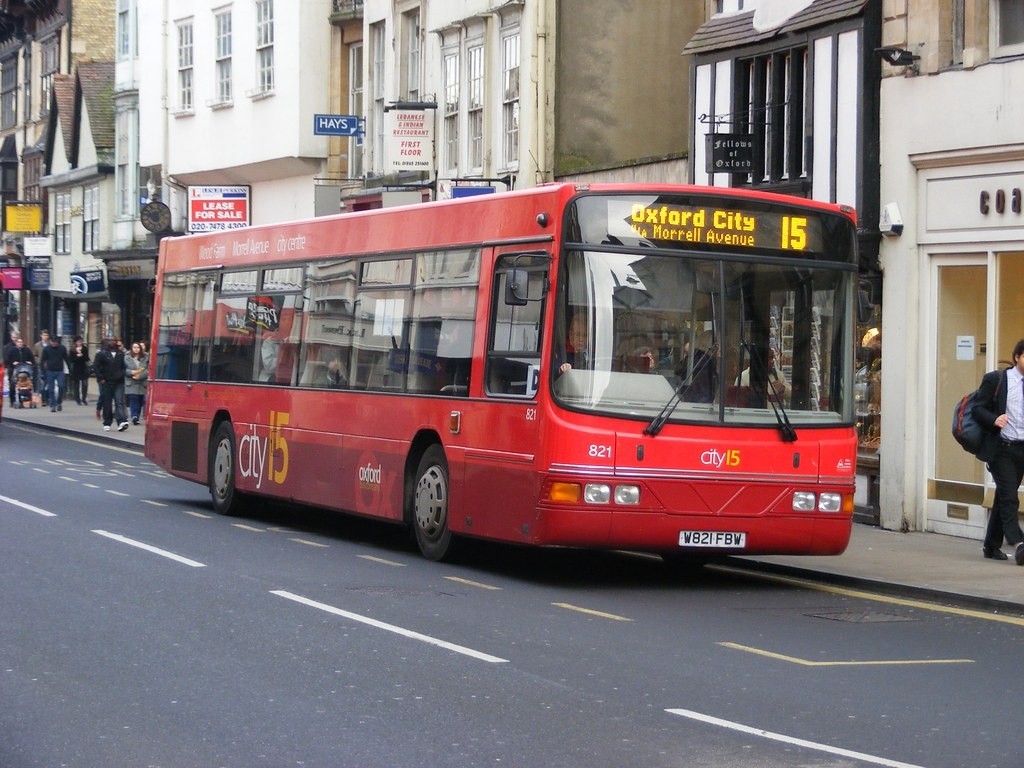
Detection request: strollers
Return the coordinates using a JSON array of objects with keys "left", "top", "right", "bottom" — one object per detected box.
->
[{"left": 12, "top": 362, "right": 37, "bottom": 409}]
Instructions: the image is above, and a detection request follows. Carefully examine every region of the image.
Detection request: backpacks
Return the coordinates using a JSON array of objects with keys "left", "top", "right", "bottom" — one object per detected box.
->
[{"left": 952, "top": 370, "right": 1003, "bottom": 462}]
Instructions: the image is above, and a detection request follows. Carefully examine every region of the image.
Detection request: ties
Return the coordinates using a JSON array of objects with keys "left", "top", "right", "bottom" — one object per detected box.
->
[{"left": 1021, "top": 378, "right": 1024, "bottom": 397}]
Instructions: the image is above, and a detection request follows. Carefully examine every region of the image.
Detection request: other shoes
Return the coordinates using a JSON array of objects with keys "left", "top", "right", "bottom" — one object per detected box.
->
[
  {"left": 983, "top": 548, "right": 1008, "bottom": 560},
  {"left": 76, "top": 400, "right": 82, "bottom": 405},
  {"left": 82, "top": 400, "right": 87, "bottom": 405},
  {"left": 9, "top": 402, "right": 13, "bottom": 407},
  {"left": 50, "top": 407, "right": 55, "bottom": 412},
  {"left": 57, "top": 403, "right": 62, "bottom": 411},
  {"left": 118, "top": 422, "right": 129, "bottom": 432},
  {"left": 96, "top": 410, "right": 101, "bottom": 419},
  {"left": 133, "top": 418, "right": 139, "bottom": 425},
  {"left": 103, "top": 425, "right": 110, "bottom": 431},
  {"left": 41, "top": 401, "right": 48, "bottom": 406},
  {"left": 1015, "top": 544, "right": 1024, "bottom": 565}
]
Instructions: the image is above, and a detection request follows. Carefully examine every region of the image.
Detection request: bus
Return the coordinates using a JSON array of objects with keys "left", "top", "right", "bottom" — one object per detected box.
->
[{"left": 144, "top": 181, "right": 858, "bottom": 566}]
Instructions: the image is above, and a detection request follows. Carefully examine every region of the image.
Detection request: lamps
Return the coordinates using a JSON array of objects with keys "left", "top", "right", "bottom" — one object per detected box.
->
[{"left": 874, "top": 45, "right": 921, "bottom": 66}]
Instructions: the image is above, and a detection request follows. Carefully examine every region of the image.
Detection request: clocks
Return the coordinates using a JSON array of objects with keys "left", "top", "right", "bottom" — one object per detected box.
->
[{"left": 141, "top": 201, "right": 171, "bottom": 233}]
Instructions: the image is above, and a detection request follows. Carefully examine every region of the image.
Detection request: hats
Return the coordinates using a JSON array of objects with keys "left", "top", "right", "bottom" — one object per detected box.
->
[
  {"left": 47, "top": 335, "right": 63, "bottom": 339},
  {"left": 18, "top": 373, "right": 27, "bottom": 381}
]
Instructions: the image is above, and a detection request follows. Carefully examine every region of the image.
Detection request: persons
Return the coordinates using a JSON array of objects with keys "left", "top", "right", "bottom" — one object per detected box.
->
[
  {"left": 733, "top": 347, "right": 792, "bottom": 409},
  {"left": 312, "top": 349, "right": 349, "bottom": 386},
  {"left": 658, "top": 329, "right": 737, "bottom": 403},
  {"left": 556, "top": 312, "right": 587, "bottom": 379},
  {"left": 93, "top": 335, "right": 149, "bottom": 431},
  {"left": 972, "top": 339, "right": 1024, "bottom": 565},
  {"left": 631, "top": 347, "right": 655, "bottom": 369},
  {"left": 3, "top": 329, "right": 90, "bottom": 412},
  {"left": 258, "top": 312, "right": 297, "bottom": 386}
]
